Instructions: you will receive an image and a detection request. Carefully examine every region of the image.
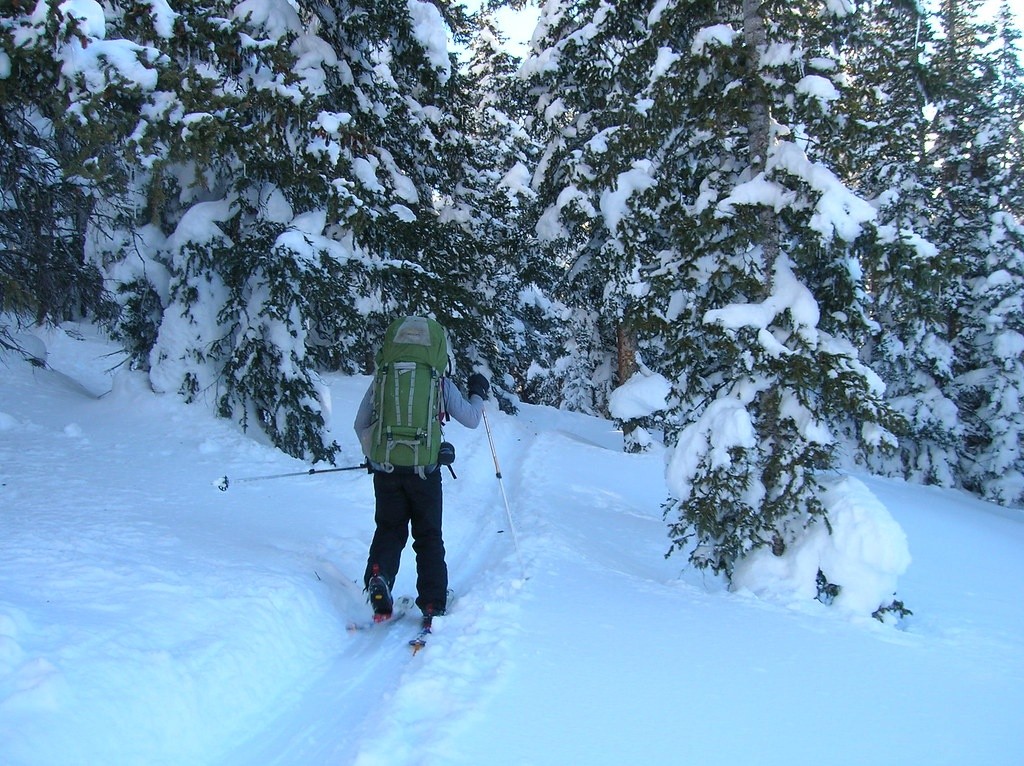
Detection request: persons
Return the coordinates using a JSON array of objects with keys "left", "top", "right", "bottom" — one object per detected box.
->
[{"left": 354, "top": 314, "right": 489, "bottom": 627}]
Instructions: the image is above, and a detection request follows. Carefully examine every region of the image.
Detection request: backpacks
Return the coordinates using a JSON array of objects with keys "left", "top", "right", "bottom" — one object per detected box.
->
[{"left": 370, "top": 316, "right": 449, "bottom": 466}]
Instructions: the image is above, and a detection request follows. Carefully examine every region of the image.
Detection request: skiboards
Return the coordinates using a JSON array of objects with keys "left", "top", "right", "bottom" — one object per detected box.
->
[{"left": 344, "top": 588, "right": 456, "bottom": 656}]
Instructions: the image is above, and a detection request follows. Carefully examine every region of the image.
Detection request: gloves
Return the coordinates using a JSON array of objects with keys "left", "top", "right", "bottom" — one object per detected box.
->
[{"left": 467, "top": 373, "right": 491, "bottom": 400}]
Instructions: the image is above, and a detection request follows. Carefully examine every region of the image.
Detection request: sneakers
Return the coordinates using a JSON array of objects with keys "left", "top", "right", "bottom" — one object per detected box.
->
[
  {"left": 368, "top": 564, "right": 393, "bottom": 622},
  {"left": 423, "top": 591, "right": 448, "bottom": 619}
]
